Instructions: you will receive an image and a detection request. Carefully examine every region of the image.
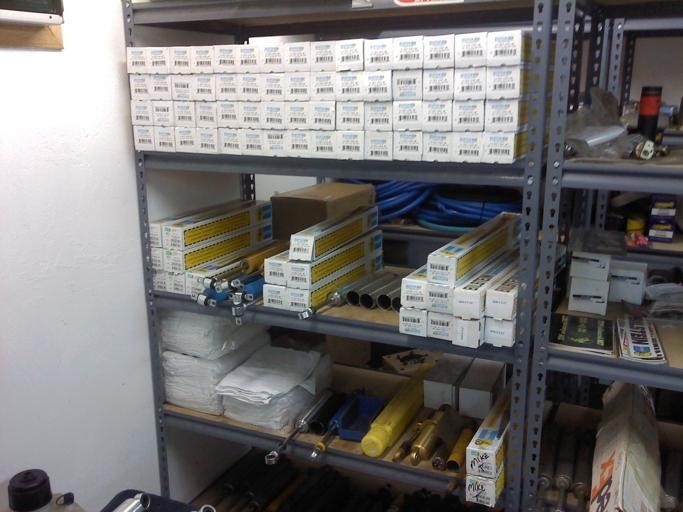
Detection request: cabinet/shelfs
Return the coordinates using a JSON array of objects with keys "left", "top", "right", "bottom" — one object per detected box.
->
[
  {"left": 522, "top": 0, "right": 683, "bottom": 512},
  {"left": 122, "top": 0, "right": 610, "bottom": 512}
]
